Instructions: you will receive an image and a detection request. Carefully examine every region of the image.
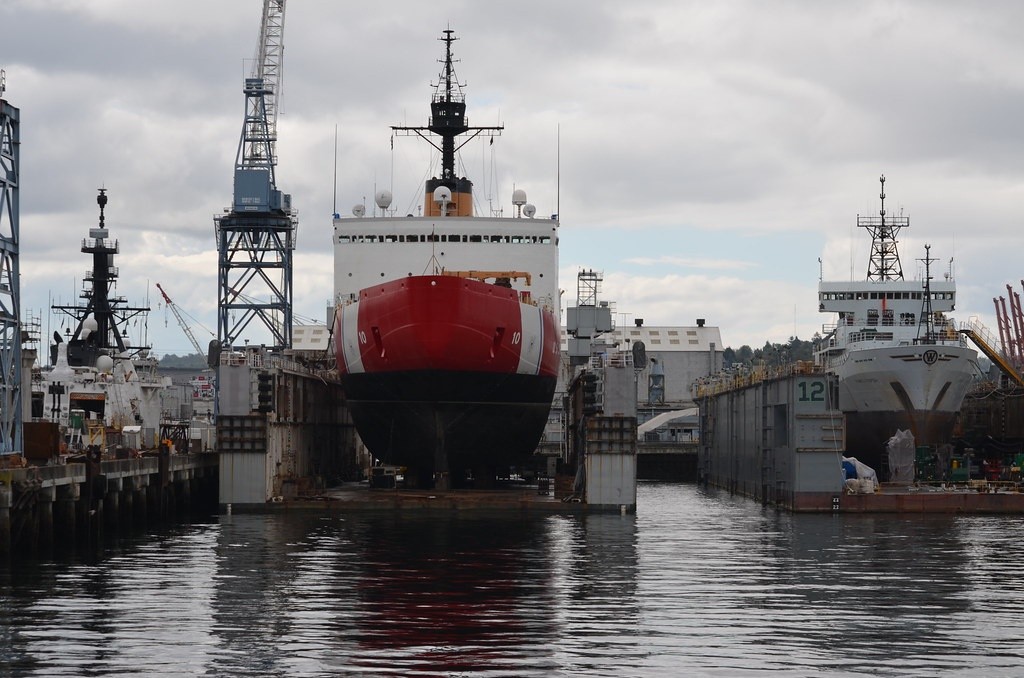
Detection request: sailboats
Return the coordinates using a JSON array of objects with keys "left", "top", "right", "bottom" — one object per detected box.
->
[
  {"left": 333, "top": 26, "right": 561, "bottom": 484},
  {"left": 39, "top": 185, "right": 171, "bottom": 451},
  {"left": 810, "top": 175, "right": 978, "bottom": 466}
]
